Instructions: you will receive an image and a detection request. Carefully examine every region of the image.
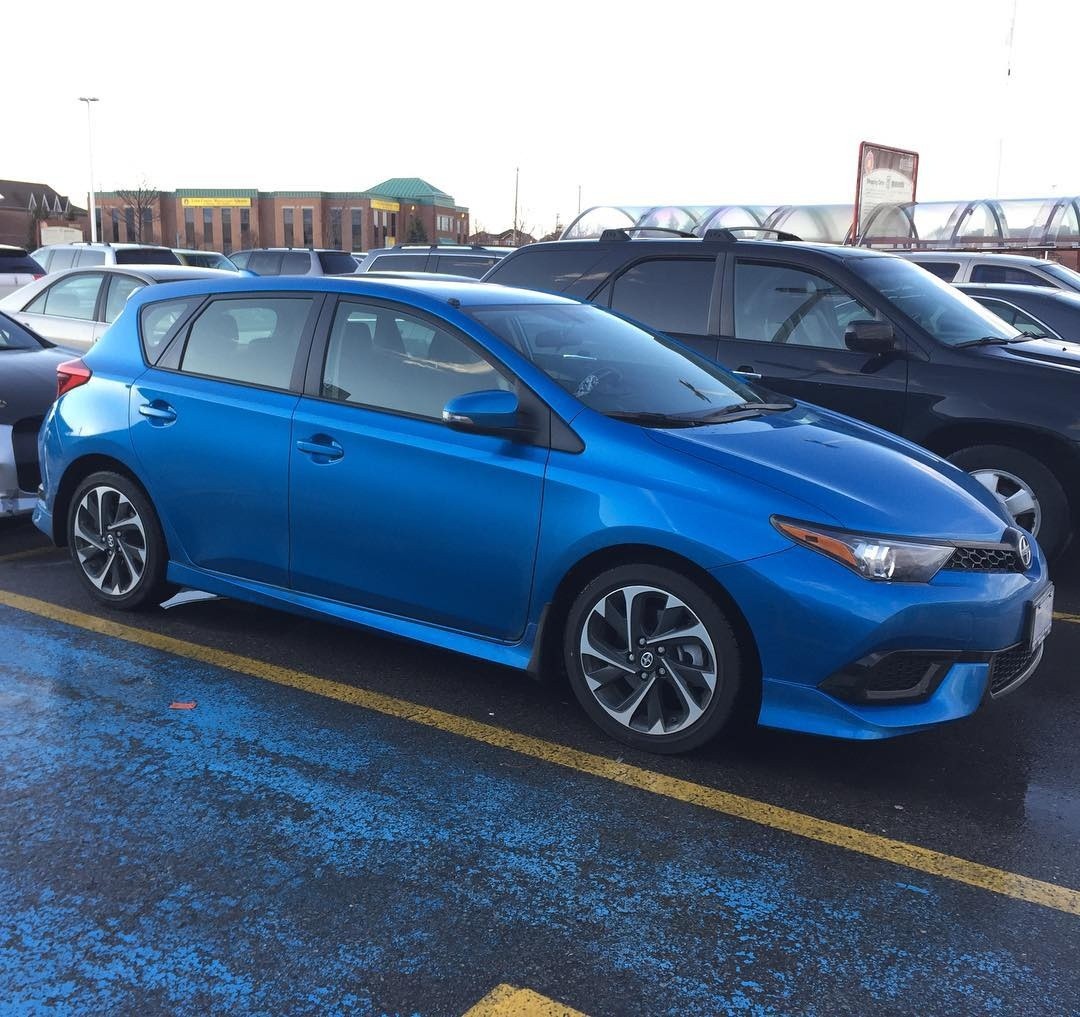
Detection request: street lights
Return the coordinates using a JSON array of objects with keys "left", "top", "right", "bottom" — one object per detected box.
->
[{"left": 77, "top": 97, "right": 102, "bottom": 245}]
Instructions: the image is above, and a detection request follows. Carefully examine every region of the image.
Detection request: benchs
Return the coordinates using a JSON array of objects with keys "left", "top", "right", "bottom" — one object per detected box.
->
[{"left": 183, "top": 317, "right": 289, "bottom": 386}]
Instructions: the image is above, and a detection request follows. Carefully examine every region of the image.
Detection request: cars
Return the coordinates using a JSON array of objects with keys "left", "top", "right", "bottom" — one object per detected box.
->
[
  {"left": 0, "top": 237, "right": 516, "bottom": 522},
  {"left": 736, "top": 245, "right": 1080, "bottom": 355},
  {"left": 27, "top": 267, "right": 1056, "bottom": 758}
]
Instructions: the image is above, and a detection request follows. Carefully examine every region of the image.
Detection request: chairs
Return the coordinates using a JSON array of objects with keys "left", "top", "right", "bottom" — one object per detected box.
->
[
  {"left": 780, "top": 279, "right": 842, "bottom": 350},
  {"left": 425, "top": 330, "right": 475, "bottom": 379},
  {"left": 52, "top": 294, "right": 82, "bottom": 314},
  {"left": 339, "top": 321, "right": 373, "bottom": 356}
]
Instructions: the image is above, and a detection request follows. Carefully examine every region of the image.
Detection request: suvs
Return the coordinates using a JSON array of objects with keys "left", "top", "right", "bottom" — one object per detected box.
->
[{"left": 462, "top": 226, "right": 1080, "bottom": 575}]
